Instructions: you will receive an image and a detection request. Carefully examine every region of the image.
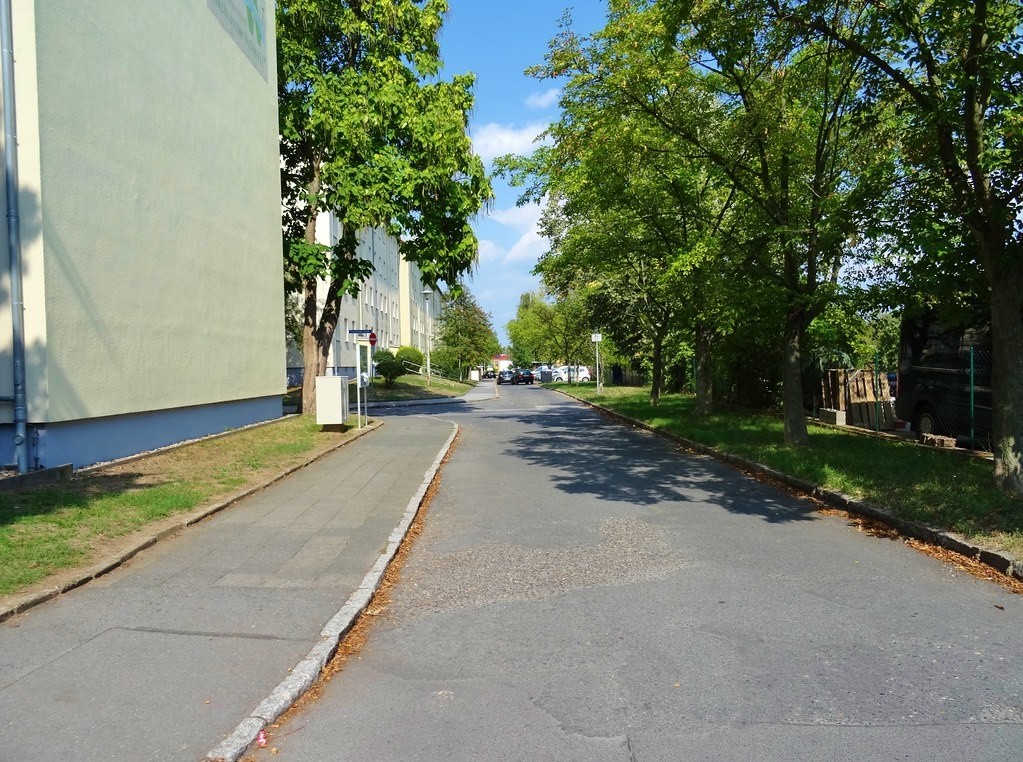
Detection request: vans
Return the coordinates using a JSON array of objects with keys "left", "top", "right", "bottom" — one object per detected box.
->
[{"left": 894, "top": 302, "right": 992, "bottom": 441}]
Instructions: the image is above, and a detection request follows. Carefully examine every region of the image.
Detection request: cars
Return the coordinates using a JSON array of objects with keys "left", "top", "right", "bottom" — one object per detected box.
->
[
  {"left": 530, "top": 365, "right": 590, "bottom": 382},
  {"left": 483, "top": 370, "right": 534, "bottom": 384}
]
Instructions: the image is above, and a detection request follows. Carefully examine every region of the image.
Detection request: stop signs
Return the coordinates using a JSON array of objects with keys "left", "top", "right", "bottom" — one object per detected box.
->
[{"left": 369, "top": 333, "right": 377, "bottom": 345}]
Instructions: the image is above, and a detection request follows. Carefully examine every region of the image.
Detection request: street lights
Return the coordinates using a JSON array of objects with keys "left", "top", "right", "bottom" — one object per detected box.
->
[{"left": 421, "top": 291, "right": 434, "bottom": 385}]
